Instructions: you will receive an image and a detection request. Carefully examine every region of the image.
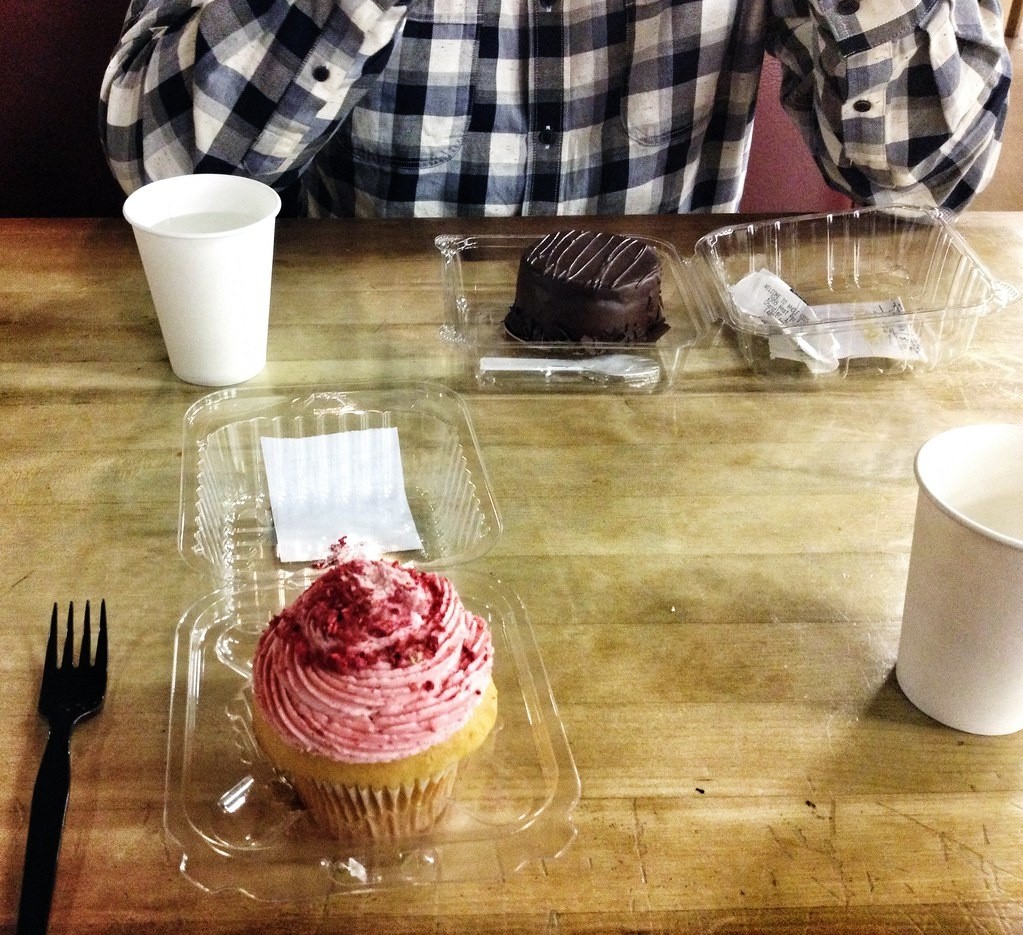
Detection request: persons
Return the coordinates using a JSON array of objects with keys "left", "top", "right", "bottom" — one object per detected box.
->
[{"left": 95, "top": 1, "right": 1016, "bottom": 227}]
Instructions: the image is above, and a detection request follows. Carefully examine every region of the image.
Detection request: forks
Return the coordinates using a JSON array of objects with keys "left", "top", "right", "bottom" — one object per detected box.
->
[{"left": 14, "top": 597, "right": 108, "bottom": 935}]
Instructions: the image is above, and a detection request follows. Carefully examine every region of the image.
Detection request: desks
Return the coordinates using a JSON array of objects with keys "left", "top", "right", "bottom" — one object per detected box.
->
[{"left": 0, "top": 212, "right": 1023, "bottom": 935}]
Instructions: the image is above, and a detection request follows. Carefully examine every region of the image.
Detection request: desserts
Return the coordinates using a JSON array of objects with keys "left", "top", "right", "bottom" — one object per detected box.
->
[
  {"left": 255, "top": 536, "right": 497, "bottom": 832},
  {"left": 505, "top": 228, "right": 666, "bottom": 343}
]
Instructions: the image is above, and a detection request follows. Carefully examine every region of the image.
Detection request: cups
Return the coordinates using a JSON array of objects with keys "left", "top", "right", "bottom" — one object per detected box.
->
[
  {"left": 894, "top": 423, "right": 1023, "bottom": 736},
  {"left": 123, "top": 173, "right": 281, "bottom": 387}
]
